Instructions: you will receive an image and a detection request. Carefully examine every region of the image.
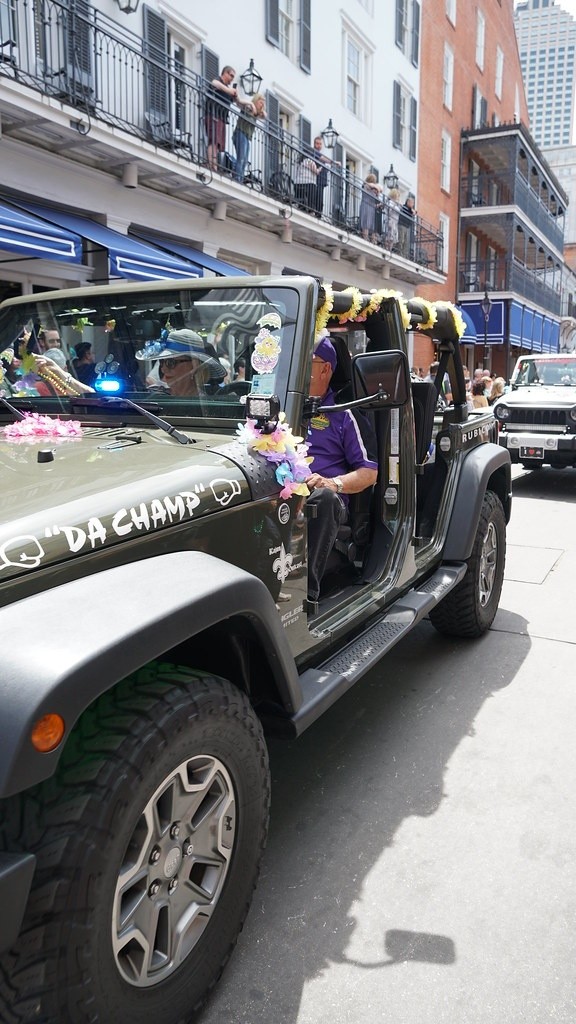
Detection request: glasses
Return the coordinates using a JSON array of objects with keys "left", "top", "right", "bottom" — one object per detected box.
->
[
  {"left": 43, "top": 338, "right": 61, "bottom": 344},
  {"left": 226, "top": 71, "right": 235, "bottom": 79},
  {"left": 159, "top": 359, "right": 194, "bottom": 370}
]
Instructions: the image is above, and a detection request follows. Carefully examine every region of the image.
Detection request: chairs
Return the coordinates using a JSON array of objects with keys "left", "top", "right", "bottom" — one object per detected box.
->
[
  {"left": 267, "top": 172, "right": 310, "bottom": 213},
  {"left": 334, "top": 205, "right": 361, "bottom": 236},
  {"left": 0, "top": 39, "right": 19, "bottom": 80},
  {"left": 226, "top": 152, "right": 264, "bottom": 191},
  {"left": 321, "top": 336, "right": 378, "bottom": 553},
  {"left": 393, "top": 242, "right": 439, "bottom": 268},
  {"left": 468, "top": 191, "right": 487, "bottom": 207},
  {"left": 39, "top": 60, "right": 103, "bottom": 115},
  {"left": 461, "top": 271, "right": 480, "bottom": 292},
  {"left": 145, "top": 112, "right": 193, "bottom": 160},
  {"left": 201, "top": 341, "right": 224, "bottom": 396}
]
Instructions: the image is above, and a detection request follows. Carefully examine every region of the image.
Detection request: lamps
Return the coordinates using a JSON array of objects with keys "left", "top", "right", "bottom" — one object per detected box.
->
[
  {"left": 213, "top": 202, "right": 227, "bottom": 221},
  {"left": 123, "top": 163, "right": 138, "bottom": 188},
  {"left": 381, "top": 265, "right": 390, "bottom": 280},
  {"left": 115, "top": 0, "right": 139, "bottom": 15},
  {"left": 282, "top": 228, "right": 293, "bottom": 243},
  {"left": 331, "top": 249, "right": 341, "bottom": 261},
  {"left": 240, "top": 59, "right": 263, "bottom": 95},
  {"left": 357, "top": 255, "right": 366, "bottom": 271},
  {"left": 322, "top": 118, "right": 339, "bottom": 149},
  {"left": 384, "top": 164, "right": 398, "bottom": 189}
]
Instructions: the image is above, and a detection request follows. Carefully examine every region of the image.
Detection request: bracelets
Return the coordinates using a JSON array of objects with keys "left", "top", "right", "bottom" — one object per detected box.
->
[{"left": 64, "top": 371, "right": 73, "bottom": 390}]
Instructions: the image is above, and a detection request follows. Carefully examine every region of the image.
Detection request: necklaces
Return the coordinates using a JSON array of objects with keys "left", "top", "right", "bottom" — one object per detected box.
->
[{"left": 39, "top": 362, "right": 80, "bottom": 397}]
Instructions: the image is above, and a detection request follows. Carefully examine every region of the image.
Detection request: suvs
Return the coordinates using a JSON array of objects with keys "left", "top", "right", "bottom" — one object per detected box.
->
[
  {"left": 468, "top": 352, "right": 576, "bottom": 471},
  {"left": 0, "top": 272, "right": 511, "bottom": 1024}
]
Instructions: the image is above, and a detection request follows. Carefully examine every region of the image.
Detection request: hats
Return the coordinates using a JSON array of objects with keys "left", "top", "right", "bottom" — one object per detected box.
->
[
  {"left": 43, "top": 348, "right": 66, "bottom": 370},
  {"left": 134, "top": 327, "right": 228, "bottom": 379},
  {"left": 313, "top": 336, "right": 337, "bottom": 373}
]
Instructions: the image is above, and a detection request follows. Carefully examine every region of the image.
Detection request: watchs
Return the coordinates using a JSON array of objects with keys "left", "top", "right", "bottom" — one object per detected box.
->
[{"left": 333, "top": 476, "right": 344, "bottom": 493}]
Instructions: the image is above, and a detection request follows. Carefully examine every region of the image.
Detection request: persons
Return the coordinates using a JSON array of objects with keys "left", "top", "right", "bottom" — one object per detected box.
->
[
  {"left": 203, "top": 65, "right": 238, "bottom": 172},
  {"left": 6, "top": 323, "right": 105, "bottom": 389},
  {"left": 359, "top": 173, "right": 416, "bottom": 258},
  {"left": 232, "top": 94, "right": 266, "bottom": 182},
  {"left": 411, "top": 361, "right": 506, "bottom": 409},
  {"left": 216, "top": 346, "right": 246, "bottom": 386},
  {"left": 135, "top": 328, "right": 228, "bottom": 399},
  {"left": 294, "top": 137, "right": 342, "bottom": 217},
  {"left": 304, "top": 333, "right": 379, "bottom": 600}
]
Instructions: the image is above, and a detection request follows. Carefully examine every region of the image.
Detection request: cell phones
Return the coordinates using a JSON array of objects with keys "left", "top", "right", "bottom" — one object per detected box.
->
[{"left": 233, "top": 84, "right": 237, "bottom": 89}]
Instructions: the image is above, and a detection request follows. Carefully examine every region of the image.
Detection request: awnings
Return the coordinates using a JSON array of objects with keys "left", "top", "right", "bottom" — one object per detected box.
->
[
  {"left": 0, "top": 194, "right": 204, "bottom": 284},
  {"left": 0, "top": 196, "right": 83, "bottom": 264},
  {"left": 129, "top": 228, "right": 256, "bottom": 277}
]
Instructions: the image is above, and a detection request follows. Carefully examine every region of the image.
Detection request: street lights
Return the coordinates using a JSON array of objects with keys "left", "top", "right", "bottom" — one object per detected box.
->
[{"left": 481, "top": 291, "right": 492, "bottom": 371}]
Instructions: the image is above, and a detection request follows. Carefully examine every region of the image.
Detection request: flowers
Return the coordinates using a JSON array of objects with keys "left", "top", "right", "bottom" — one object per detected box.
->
[
  {"left": 105, "top": 319, "right": 116, "bottom": 332},
  {"left": 314, "top": 283, "right": 467, "bottom": 339},
  {"left": 235, "top": 411, "right": 314, "bottom": 500},
  {"left": 0, "top": 410, "right": 84, "bottom": 439}
]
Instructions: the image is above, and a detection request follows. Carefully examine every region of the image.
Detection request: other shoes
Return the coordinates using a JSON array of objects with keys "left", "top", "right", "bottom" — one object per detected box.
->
[
  {"left": 277, "top": 592, "right": 291, "bottom": 601},
  {"left": 275, "top": 603, "right": 280, "bottom": 612}
]
